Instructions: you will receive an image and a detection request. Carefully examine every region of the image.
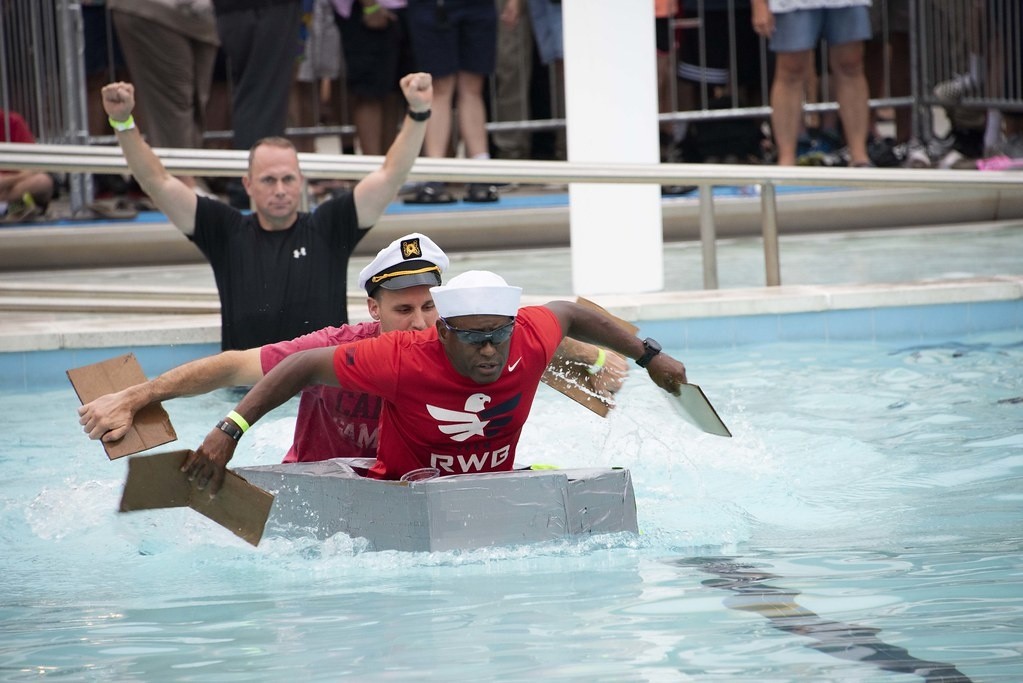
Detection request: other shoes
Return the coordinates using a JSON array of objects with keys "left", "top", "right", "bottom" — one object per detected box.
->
[{"left": 770, "top": 138, "right": 1023, "bottom": 177}]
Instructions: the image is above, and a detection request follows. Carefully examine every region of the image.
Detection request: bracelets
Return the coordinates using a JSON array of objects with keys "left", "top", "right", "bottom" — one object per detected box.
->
[
  {"left": 109, "top": 114, "right": 135, "bottom": 132},
  {"left": 407, "top": 108, "right": 431, "bottom": 122},
  {"left": 216, "top": 420, "right": 242, "bottom": 440},
  {"left": 226, "top": 410, "right": 249, "bottom": 434},
  {"left": 363, "top": 3, "right": 381, "bottom": 15},
  {"left": 585, "top": 348, "right": 606, "bottom": 375}
]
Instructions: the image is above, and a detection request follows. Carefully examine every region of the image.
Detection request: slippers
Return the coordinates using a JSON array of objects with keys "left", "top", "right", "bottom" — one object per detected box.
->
[
  {"left": 404, "top": 179, "right": 500, "bottom": 207},
  {"left": 53, "top": 196, "right": 136, "bottom": 222}
]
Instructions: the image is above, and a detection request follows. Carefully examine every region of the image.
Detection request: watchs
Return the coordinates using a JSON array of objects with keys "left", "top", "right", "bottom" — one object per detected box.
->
[{"left": 636, "top": 337, "right": 662, "bottom": 368}]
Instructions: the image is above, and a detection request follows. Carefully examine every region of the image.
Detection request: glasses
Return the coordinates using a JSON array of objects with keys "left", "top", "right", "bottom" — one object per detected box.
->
[{"left": 440, "top": 314, "right": 515, "bottom": 345}]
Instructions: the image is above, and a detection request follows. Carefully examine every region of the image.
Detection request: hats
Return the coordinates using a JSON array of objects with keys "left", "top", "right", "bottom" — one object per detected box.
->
[
  {"left": 429, "top": 270, "right": 521, "bottom": 318},
  {"left": 358, "top": 232, "right": 449, "bottom": 293}
]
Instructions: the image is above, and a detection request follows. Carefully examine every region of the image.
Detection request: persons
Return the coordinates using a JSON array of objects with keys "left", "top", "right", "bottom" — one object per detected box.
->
[
  {"left": 78, "top": 73, "right": 687, "bottom": 499},
  {"left": 0, "top": 109, "right": 60, "bottom": 223},
  {"left": 655, "top": 0, "right": 1023, "bottom": 195},
  {"left": 81, "top": 0, "right": 562, "bottom": 208}
]
